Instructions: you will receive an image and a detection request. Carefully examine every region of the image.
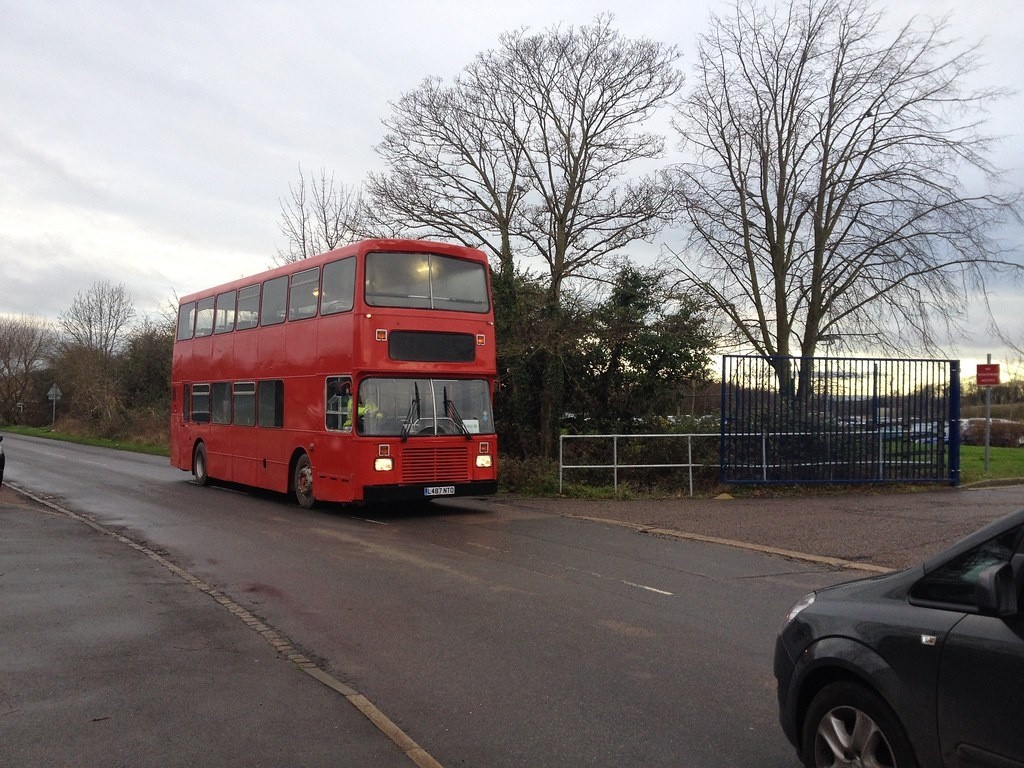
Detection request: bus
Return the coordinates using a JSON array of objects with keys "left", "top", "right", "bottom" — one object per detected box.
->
[{"left": 170, "top": 238, "right": 499, "bottom": 510}]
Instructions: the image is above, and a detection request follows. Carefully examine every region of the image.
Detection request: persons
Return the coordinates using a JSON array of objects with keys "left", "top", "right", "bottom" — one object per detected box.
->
[{"left": 343, "top": 383, "right": 382, "bottom": 432}]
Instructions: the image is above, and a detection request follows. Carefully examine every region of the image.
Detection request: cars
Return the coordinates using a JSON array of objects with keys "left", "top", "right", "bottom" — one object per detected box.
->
[
  {"left": 560, "top": 411, "right": 1024, "bottom": 445},
  {"left": 772, "top": 507, "right": 1024, "bottom": 767}
]
